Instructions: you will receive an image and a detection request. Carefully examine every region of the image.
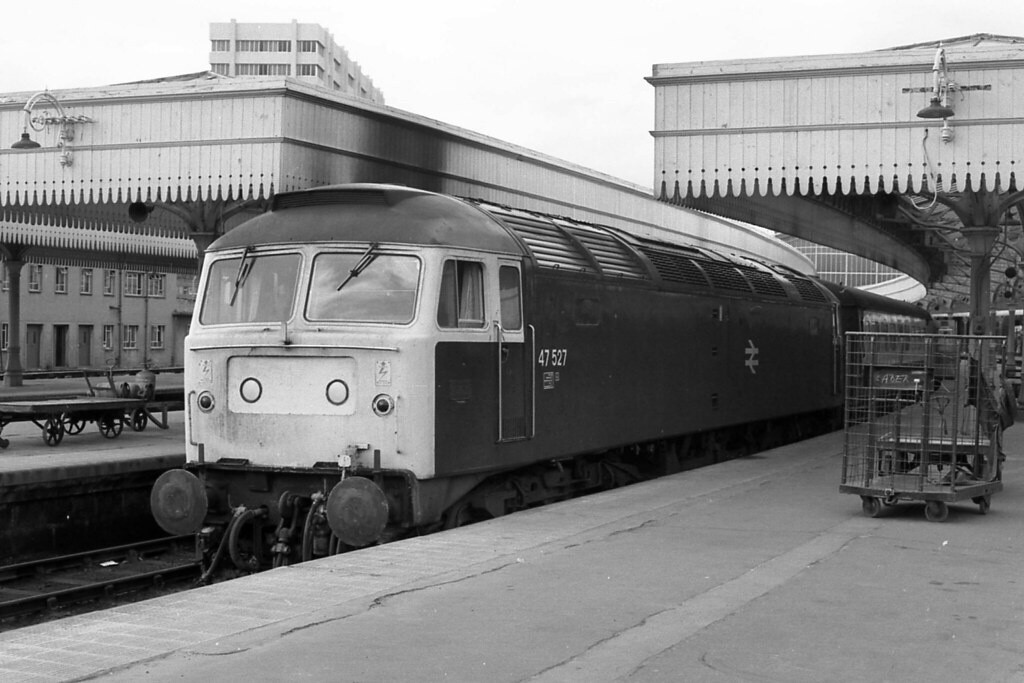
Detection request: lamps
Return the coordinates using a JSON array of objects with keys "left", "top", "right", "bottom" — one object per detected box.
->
[
  {"left": 10, "top": 91, "right": 92, "bottom": 168},
  {"left": 915, "top": 47, "right": 958, "bottom": 143}
]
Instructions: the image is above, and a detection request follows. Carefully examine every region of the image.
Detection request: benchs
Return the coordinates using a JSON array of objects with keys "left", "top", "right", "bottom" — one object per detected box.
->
[
  {"left": 93, "top": 383, "right": 184, "bottom": 433},
  {"left": 0, "top": 394, "right": 148, "bottom": 447}
]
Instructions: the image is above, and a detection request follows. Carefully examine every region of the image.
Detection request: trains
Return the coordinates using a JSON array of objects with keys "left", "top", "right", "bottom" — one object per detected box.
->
[{"left": 147, "top": 182, "right": 1024, "bottom": 596}]
